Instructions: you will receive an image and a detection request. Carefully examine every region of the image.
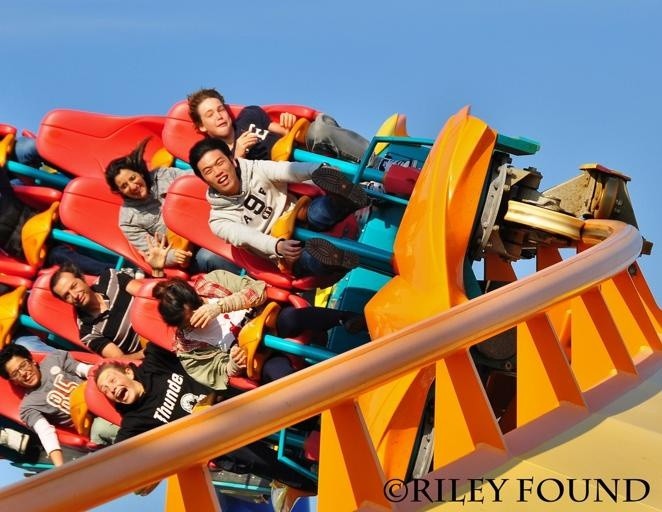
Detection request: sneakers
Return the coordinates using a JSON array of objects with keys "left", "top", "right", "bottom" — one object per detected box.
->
[
  {"left": 312, "top": 168, "right": 370, "bottom": 207},
  {"left": 304, "top": 238, "right": 359, "bottom": 269}
]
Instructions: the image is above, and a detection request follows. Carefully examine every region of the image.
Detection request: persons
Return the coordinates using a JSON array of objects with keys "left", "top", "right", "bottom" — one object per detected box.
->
[{"left": 1, "top": 86, "right": 373, "bottom": 494}]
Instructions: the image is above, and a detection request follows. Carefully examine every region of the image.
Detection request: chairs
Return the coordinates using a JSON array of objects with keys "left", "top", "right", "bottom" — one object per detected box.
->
[{"left": 0, "top": 99, "right": 430, "bottom": 511}]
